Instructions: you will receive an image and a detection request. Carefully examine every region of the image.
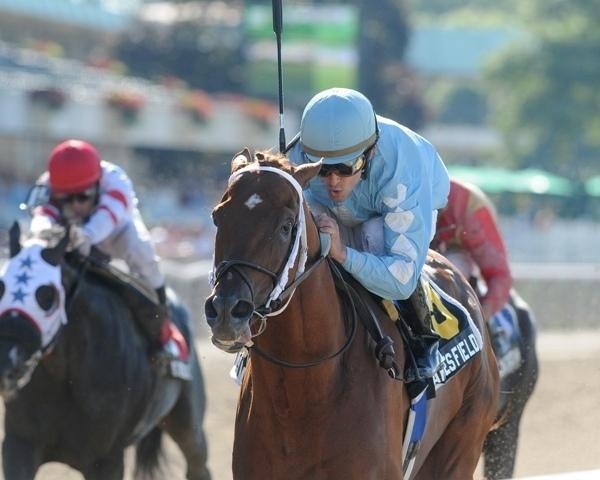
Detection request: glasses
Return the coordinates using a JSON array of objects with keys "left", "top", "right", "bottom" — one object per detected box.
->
[
  {"left": 53, "top": 192, "right": 98, "bottom": 206},
  {"left": 306, "top": 159, "right": 366, "bottom": 179}
]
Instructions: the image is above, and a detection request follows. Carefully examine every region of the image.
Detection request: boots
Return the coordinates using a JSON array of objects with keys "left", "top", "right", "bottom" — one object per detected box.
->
[{"left": 387, "top": 276, "right": 446, "bottom": 382}]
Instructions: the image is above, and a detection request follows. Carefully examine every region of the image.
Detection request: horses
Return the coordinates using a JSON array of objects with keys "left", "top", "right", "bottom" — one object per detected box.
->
[
  {"left": 0, "top": 219, "right": 211, "bottom": 480},
  {"left": 472, "top": 278, "right": 540, "bottom": 479},
  {"left": 204, "top": 147, "right": 502, "bottom": 479}
]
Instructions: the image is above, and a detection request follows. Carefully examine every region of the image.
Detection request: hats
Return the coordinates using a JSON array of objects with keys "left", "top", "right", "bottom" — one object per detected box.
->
[{"left": 45, "top": 139, "right": 104, "bottom": 191}]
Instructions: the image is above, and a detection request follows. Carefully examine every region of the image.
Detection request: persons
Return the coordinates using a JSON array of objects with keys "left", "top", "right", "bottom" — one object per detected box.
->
[
  {"left": 281, "top": 84, "right": 452, "bottom": 303},
  {"left": 432, "top": 178, "right": 515, "bottom": 323},
  {"left": 26, "top": 136, "right": 191, "bottom": 363}
]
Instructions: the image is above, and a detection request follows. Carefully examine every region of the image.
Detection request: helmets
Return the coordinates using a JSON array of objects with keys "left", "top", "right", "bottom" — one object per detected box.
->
[{"left": 298, "top": 86, "right": 383, "bottom": 167}]
